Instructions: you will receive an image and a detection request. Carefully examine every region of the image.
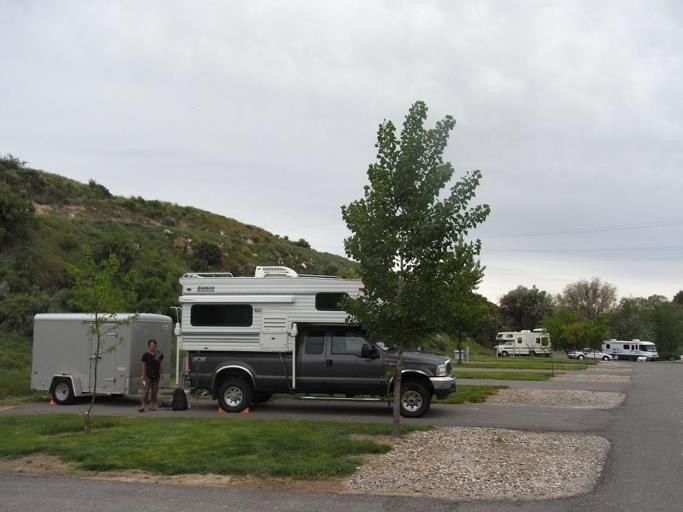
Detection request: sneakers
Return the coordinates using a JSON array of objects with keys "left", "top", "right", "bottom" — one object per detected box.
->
[
  {"left": 138, "top": 408, "right": 144, "bottom": 412},
  {"left": 149, "top": 408, "right": 155, "bottom": 410}
]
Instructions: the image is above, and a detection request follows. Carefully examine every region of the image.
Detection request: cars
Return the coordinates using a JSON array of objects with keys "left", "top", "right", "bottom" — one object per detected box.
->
[{"left": 568, "top": 347, "right": 613, "bottom": 360}]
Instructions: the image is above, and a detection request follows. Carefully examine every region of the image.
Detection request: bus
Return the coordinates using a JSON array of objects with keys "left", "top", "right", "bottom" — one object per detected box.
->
[
  {"left": 601, "top": 338, "right": 660, "bottom": 360},
  {"left": 494, "top": 328, "right": 551, "bottom": 357}
]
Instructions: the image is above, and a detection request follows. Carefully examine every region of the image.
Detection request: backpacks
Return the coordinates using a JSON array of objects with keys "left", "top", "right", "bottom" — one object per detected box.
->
[{"left": 171, "top": 388, "right": 187, "bottom": 410}]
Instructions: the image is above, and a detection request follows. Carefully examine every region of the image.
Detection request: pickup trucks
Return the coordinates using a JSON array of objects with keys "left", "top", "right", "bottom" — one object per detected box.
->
[{"left": 183, "top": 321, "right": 456, "bottom": 418}]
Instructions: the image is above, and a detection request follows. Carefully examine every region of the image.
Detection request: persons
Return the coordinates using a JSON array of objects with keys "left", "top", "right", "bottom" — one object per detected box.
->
[{"left": 138, "top": 339, "right": 165, "bottom": 412}]
